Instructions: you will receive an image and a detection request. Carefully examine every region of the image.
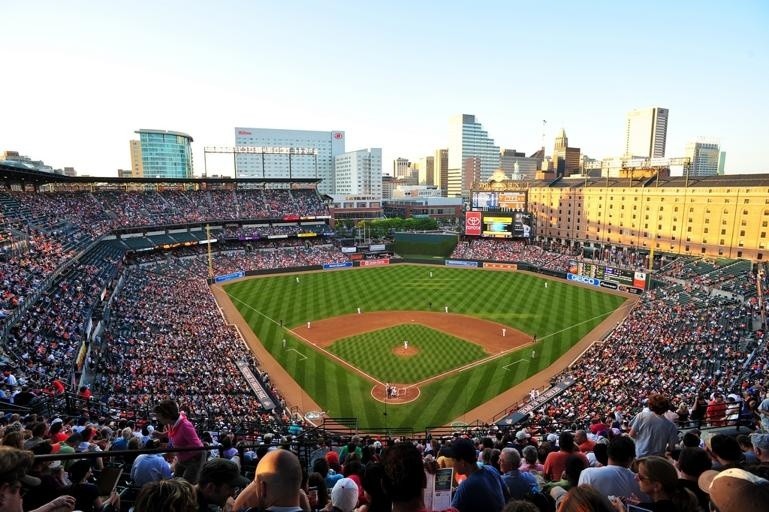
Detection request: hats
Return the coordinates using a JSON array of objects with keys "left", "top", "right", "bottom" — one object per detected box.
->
[
  {"left": 511, "top": 422, "right": 634, "bottom": 450},
  {"left": 331, "top": 477, "right": 359, "bottom": 511},
  {"left": 440, "top": 436, "right": 477, "bottom": 461},
  {"left": 696, "top": 465, "right": 769, "bottom": 511},
  {"left": 200, "top": 457, "right": 251, "bottom": 486},
  {"left": 261, "top": 430, "right": 395, "bottom": 467}
]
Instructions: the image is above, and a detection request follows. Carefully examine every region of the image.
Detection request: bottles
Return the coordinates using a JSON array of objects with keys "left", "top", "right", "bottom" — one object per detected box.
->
[{"left": 513, "top": 214, "right": 524, "bottom": 236}]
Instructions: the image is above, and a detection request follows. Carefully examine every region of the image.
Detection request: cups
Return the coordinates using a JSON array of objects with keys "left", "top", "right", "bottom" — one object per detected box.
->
[
  {"left": 522, "top": 224, "right": 531, "bottom": 232},
  {"left": 305, "top": 485, "right": 320, "bottom": 505}
]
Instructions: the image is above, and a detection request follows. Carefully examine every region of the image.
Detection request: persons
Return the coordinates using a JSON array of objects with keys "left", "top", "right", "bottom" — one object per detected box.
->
[
  {"left": 583, "top": 293, "right": 769, "bottom": 355},
  {"left": 359, "top": 353, "right": 769, "bottom": 512},
  {"left": 451, "top": 230, "right": 769, "bottom": 293},
  {"left": 0, "top": 153, "right": 361, "bottom": 512}
]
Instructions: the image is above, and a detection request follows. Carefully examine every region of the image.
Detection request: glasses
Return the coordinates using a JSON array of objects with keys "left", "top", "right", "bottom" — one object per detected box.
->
[{"left": 638, "top": 473, "right": 656, "bottom": 482}]
